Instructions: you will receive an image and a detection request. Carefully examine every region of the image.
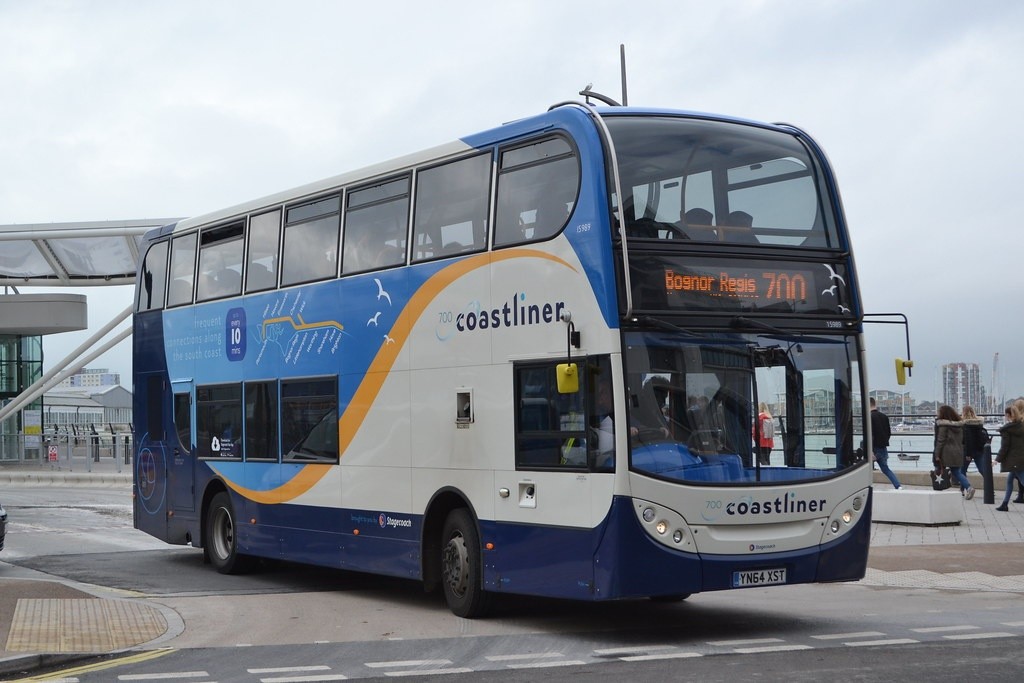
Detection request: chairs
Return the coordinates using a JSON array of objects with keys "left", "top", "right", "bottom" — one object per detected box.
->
[{"left": 166, "top": 204, "right": 760, "bottom": 305}]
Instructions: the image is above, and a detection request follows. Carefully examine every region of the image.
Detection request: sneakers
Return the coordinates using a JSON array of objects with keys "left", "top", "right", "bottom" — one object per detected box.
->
[{"left": 965, "top": 486, "right": 975, "bottom": 500}]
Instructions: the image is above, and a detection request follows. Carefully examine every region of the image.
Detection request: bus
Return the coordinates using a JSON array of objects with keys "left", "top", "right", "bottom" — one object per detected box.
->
[{"left": 132, "top": 99, "right": 915, "bottom": 620}]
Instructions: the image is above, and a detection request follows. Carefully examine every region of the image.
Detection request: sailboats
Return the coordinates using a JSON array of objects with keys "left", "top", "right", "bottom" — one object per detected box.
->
[
  {"left": 803, "top": 388, "right": 836, "bottom": 435},
  {"left": 885, "top": 383, "right": 936, "bottom": 434},
  {"left": 981, "top": 391, "right": 1014, "bottom": 436}
]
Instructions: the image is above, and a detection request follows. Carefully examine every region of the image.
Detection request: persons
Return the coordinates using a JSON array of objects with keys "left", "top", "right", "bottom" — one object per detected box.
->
[
  {"left": 561, "top": 377, "right": 727, "bottom": 469},
  {"left": 534, "top": 184, "right": 568, "bottom": 237},
  {"left": 752, "top": 401, "right": 774, "bottom": 465},
  {"left": 994, "top": 399, "right": 1024, "bottom": 512},
  {"left": 933, "top": 405, "right": 989, "bottom": 501},
  {"left": 868, "top": 397, "right": 903, "bottom": 492}
]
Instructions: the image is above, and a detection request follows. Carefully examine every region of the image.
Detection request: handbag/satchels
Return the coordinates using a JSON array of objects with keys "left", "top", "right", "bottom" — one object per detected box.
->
[{"left": 930, "top": 458, "right": 951, "bottom": 490}]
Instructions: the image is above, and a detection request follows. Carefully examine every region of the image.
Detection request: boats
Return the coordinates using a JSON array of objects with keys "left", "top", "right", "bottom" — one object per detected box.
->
[{"left": 896, "top": 439, "right": 920, "bottom": 461}]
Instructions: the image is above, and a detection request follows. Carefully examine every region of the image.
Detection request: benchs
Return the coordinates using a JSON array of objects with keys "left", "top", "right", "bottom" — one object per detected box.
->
[{"left": 872, "top": 491, "right": 964, "bottom": 524}]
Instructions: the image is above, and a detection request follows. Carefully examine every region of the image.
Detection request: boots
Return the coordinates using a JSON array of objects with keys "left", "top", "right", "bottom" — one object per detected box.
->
[
  {"left": 1013, "top": 480, "right": 1024, "bottom": 503},
  {"left": 996, "top": 501, "right": 1008, "bottom": 511}
]
